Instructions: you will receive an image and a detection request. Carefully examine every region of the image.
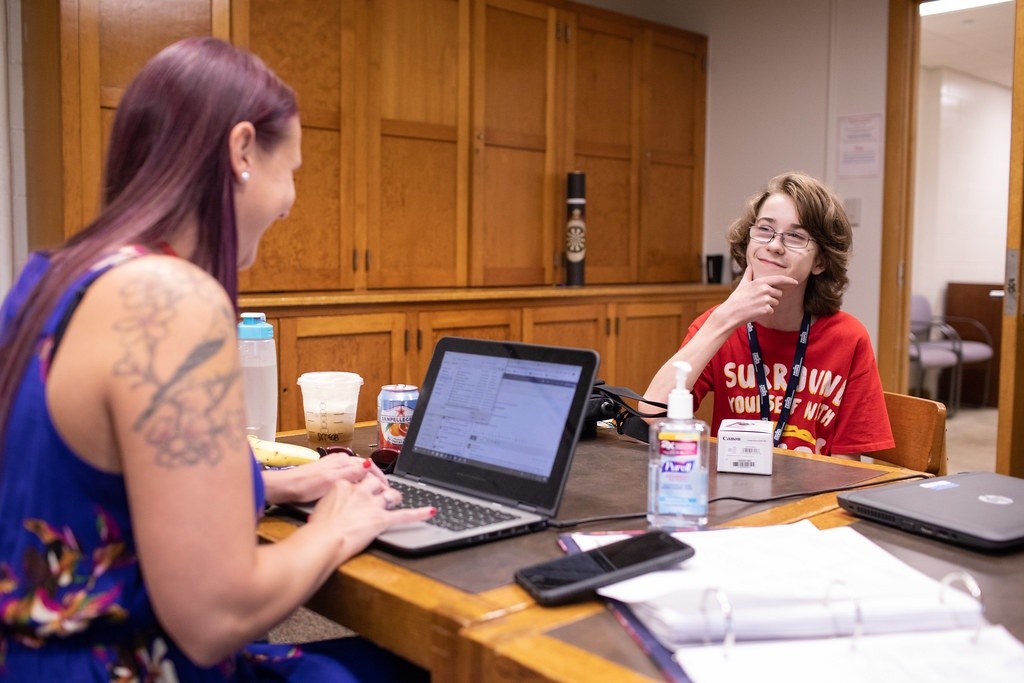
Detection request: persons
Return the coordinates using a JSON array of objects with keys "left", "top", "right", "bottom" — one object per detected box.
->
[
  {"left": 638, "top": 172, "right": 895, "bottom": 463},
  {"left": 0, "top": 38, "right": 437, "bottom": 683}
]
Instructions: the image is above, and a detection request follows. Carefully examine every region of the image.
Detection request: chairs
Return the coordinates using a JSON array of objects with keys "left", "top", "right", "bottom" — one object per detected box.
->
[
  {"left": 906, "top": 297, "right": 995, "bottom": 412},
  {"left": 859, "top": 391, "right": 949, "bottom": 477}
]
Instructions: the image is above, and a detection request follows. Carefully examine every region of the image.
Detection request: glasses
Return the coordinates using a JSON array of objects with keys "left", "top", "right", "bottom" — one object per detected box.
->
[{"left": 749, "top": 224, "right": 816, "bottom": 249}]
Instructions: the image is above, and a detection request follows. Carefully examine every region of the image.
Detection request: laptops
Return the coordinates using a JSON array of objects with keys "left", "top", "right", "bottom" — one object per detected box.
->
[
  {"left": 278, "top": 336, "right": 600, "bottom": 558},
  {"left": 836, "top": 469, "right": 1024, "bottom": 551}
]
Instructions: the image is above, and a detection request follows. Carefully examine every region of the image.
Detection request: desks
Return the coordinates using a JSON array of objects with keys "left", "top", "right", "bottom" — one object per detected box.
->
[{"left": 257, "top": 418, "right": 1024, "bottom": 683}]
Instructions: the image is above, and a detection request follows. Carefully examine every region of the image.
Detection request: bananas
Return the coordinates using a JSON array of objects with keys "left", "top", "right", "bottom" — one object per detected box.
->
[{"left": 246, "top": 435, "right": 321, "bottom": 468}]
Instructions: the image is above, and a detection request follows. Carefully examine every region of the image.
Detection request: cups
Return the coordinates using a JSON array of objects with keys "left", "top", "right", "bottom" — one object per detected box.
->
[
  {"left": 297, "top": 371, "right": 364, "bottom": 453},
  {"left": 705, "top": 256, "right": 724, "bottom": 282}
]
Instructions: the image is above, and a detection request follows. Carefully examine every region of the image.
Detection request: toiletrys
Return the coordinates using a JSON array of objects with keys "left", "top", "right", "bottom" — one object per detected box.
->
[{"left": 646, "top": 359, "right": 712, "bottom": 527}]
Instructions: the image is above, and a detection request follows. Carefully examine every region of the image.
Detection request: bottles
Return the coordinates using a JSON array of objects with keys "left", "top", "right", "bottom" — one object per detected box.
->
[{"left": 236, "top": 311, "right": 278, "bottom": 443}]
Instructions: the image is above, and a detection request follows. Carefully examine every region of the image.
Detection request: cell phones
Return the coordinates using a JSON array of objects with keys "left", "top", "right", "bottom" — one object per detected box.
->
[{"left": 512, "top": 529, "right": 695, "bottom": 605}]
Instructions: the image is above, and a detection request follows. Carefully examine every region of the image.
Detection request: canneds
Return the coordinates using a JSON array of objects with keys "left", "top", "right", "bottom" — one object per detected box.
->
[{"left": 377, "top": 383, "right": 419, "bottom": 464}]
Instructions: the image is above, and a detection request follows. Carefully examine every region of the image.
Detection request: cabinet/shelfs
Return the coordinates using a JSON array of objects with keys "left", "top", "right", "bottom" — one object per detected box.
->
[
  {"left": 947, "top": 283, "right": 1005, "bottom": 409},
  {"left": 20, "top": 0, "right": 737, "bottom": 432}
]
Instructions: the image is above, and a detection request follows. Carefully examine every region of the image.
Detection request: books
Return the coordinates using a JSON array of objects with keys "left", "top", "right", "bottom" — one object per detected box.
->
[{"left": 556, "top": 518, "right": 1024, "bottom": 683}]
